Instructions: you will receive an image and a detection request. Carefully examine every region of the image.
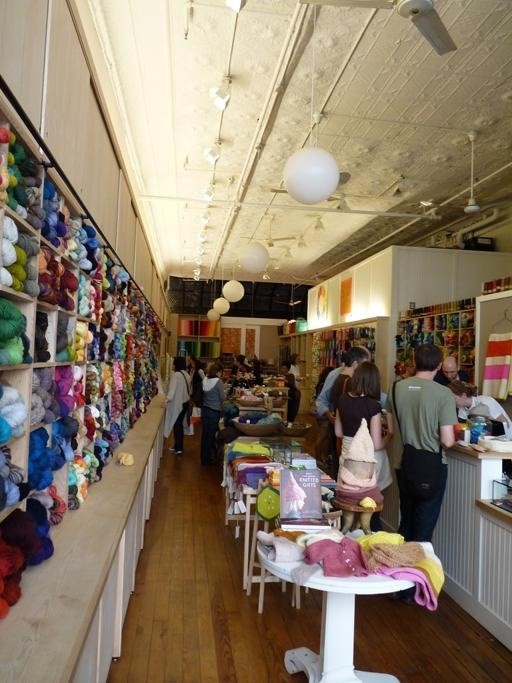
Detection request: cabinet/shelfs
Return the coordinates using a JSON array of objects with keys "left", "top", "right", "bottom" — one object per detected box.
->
[{"left": 396, "top": 309, "right": 475, "bottom": 384}]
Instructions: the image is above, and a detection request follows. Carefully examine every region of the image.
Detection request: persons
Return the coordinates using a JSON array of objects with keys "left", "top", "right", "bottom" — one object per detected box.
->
[
  {"left": 334, "top": 360, "right": 395, "bottom": 535},
  {"left": 163, "top": 355, "right": 192, "bottom": 456},
  {"left": 385, "top": 342, "right": 458, "bottom": 606},
  {"left": 450, "top": 380, "right": 511, "bottom": 442},
  {"left": 182, "top": 345, "right": 387, "bottom": 468},
  {"left": 434, "top": 354, "right": 469, "bottom": 388},
  {"left": 196, "top": 362, "right": 230, "bottom": 471}
]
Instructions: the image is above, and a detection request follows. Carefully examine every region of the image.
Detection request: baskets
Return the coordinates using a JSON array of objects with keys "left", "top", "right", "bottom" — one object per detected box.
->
[
  {"left": 273, "top": 400, "right": 286, "bottom": 407},
  {"left": 236, "top": 398, "right": 263, "bottom": 406},
  {"left": 281, "top": 421, "right": 312, "bottom": 437},
  {"left": 235, "top": 417, "right": 281, "bottom": 436}
]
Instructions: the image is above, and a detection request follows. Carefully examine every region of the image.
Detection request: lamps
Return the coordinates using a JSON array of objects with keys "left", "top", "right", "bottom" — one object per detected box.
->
[
  {"left": 284, "top": 3, "right": 340, "bottom": 204},
  {"left": 207, "top": 185, "right": 270, "bottom": 321}
]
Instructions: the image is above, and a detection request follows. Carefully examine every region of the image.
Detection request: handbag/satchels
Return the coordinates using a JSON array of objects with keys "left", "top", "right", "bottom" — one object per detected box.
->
[
  {"left": 411, "top": 479, "right": 431, "bottom": 501},
  {"left": 187, "top": 400, "right": 193, "bottom": 416}
]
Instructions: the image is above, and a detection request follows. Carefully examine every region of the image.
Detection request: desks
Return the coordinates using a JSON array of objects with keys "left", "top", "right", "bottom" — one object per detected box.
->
[{"left": 257, "top": 532, "right": 415, "bottom": 683}]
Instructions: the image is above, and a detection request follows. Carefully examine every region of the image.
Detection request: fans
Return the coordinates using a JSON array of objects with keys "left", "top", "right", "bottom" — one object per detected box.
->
[
  {"left": 429, "top": 128, "right": 512, "bottom": 212},
  {"left": 300, "top": 0, "right": 459, "bottom": 55},
  {"left": 270, "top": 111, "right": 350, "bottom": 201}
]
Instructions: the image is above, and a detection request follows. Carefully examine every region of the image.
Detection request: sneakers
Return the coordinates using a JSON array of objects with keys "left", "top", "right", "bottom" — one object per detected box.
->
[
  {"left": 175, "top": 450, "right": 182, "bottom": 454},
  {"left": 168, "top": 447, "right": 175, "bottom": 451}
]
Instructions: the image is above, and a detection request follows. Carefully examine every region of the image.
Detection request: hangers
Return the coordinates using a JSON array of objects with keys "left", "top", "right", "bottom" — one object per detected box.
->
[{"left": 490, "top": 307, "right": 512, "bottom": 337}]
